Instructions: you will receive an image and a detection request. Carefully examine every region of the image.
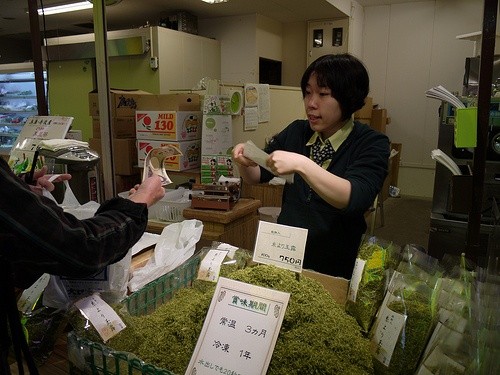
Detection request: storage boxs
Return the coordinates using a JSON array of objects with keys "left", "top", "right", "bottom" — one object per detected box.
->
[
  {"left": 155, "top": 189, "right": 196, "bottom": 222},
  {"left": 89, "top": 138, "right": 140, "bottom": 176},
  {"left": 354, "top": 97, "right": 403, "bottom": 204},
  {"left": 87, "top": 86, "right": 153, "bottom": 117},
  {"left": 159, "top": 12, "right": 199, "bottom": 35},
  {"left": 137, "top": 139, "right": 202, "bottom": 171},
  {"left": 428, "top": 210, "right": 499, "bottom": 262},
  {"left": 202, "top": 114, "right": 232, "bottom": 155},
  {"left": 200, "top": 95, "right": 233, "bottom": 115},
  {"left": 117, "top": 189, "right": 159, "bottom": 219},
  {"left": 200, "top": 169, "right": 232, "bottom": 185},
  {"left": 200, "top": 155, "right": 232, "bottom": 169},
  {"left": 134, "top": 92, "right": 201, "bottom": 111},
  {"left": 135, "top": 111, "right": 202, "bottom": 142},
  {"left": 91, "top": 117, "right": 137, "bottom": 138}
]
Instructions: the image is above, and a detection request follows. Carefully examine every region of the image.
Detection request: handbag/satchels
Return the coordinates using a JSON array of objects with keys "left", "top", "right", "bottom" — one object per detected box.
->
[{"left": 41, "top": 173, "right": 135, "bottom": 310}]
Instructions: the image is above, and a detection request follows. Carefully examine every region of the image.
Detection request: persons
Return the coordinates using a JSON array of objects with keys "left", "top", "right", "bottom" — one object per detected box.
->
[
  {"left": 0, "top": 154, "right": 166, "bottom": 375},
  {"left": 231, "top": 53, "right": 392, "bottom": 279}
]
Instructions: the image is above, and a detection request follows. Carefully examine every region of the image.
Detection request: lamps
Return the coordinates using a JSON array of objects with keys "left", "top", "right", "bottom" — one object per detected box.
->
[{"left": 25, "top": 0, "right": 93, "bottom": 16}]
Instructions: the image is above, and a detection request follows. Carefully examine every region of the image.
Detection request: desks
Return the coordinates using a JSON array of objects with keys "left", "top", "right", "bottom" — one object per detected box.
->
[
  {"left": 141, "top": 197, "right": 261, "bottom": 254},
  {"left": 241, "top": 180, "right": 284, "bottom": 207}
]
